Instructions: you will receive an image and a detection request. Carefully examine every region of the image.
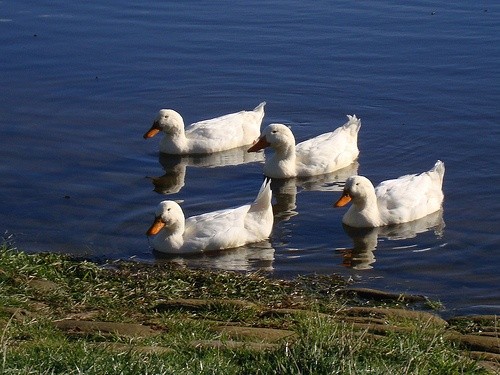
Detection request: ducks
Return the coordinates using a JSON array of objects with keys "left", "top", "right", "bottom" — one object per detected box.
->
[
  {"left": 332, "top": 158, "right": 445, "bottom": 229},
  {"left": 144, "top": 144, "right": 267, "bottom": 195},
  {"left": 246, "top": 113, "right": 362, "bottom": 179},
  {"left": 141, "top": 101, "right": 266, "bottom": 155},
  {"left": 145, "top": 177, "right": 275, "bottom": 254}
]
